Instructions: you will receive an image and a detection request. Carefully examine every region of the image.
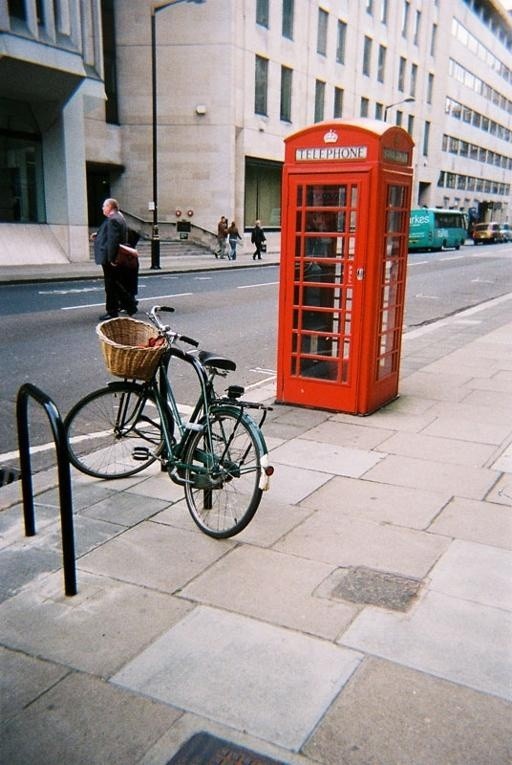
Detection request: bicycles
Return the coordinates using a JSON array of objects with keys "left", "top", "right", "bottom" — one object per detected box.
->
[{"left": 61, "top": 304, "right": 274, "bottom": 541}]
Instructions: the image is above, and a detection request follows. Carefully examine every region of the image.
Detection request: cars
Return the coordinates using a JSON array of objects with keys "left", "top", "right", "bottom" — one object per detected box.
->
[{"left": 472, "top": 221, "right": 512, "bottom": 245}]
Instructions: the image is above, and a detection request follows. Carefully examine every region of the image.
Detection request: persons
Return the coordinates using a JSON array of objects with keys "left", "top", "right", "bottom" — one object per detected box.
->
[
  {"left": 250, "top": 219, "right": 267, "bottom": 260},
  {"left": 214, "top": 215, "right": 228, "bottom": 260},
  {"left": 88, "top": 197, "right": 140, "bottom": 322},
  {"left": 224, "top": 221, "right": 243, "bottom": 261}
]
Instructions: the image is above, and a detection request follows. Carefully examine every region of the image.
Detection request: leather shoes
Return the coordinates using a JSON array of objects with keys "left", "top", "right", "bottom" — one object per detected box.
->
[{"left": 100, "top": 314, "right": 113, "bottom": 320}]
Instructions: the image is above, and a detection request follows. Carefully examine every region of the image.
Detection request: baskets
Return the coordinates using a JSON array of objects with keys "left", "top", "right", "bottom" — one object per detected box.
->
[{"left": 96, "top": 316, "right": 168, "bottom": 379}]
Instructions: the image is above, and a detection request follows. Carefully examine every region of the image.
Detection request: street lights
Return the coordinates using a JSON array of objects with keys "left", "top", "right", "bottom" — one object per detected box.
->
[
  {"left": 384, "top": 97, "right": 416, "bottom": 123},
  {"left": 149, "top": 0, "right": 207, "bottom": 271}
]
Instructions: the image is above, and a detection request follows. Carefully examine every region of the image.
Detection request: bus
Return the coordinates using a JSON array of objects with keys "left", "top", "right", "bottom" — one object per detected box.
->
[{"left": 407, "top": 207, "right": 468, "bottom": 253}]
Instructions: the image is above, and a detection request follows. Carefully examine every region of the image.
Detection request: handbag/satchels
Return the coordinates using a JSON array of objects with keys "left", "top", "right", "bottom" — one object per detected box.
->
[{"left": 114, "top": 244, "right": 138, "bottom": 269}]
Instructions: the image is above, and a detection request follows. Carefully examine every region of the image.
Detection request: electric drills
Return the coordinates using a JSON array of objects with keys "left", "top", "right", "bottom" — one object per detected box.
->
[{"left": 300, "top": 227, "right": 329, "bottom": 272}]
